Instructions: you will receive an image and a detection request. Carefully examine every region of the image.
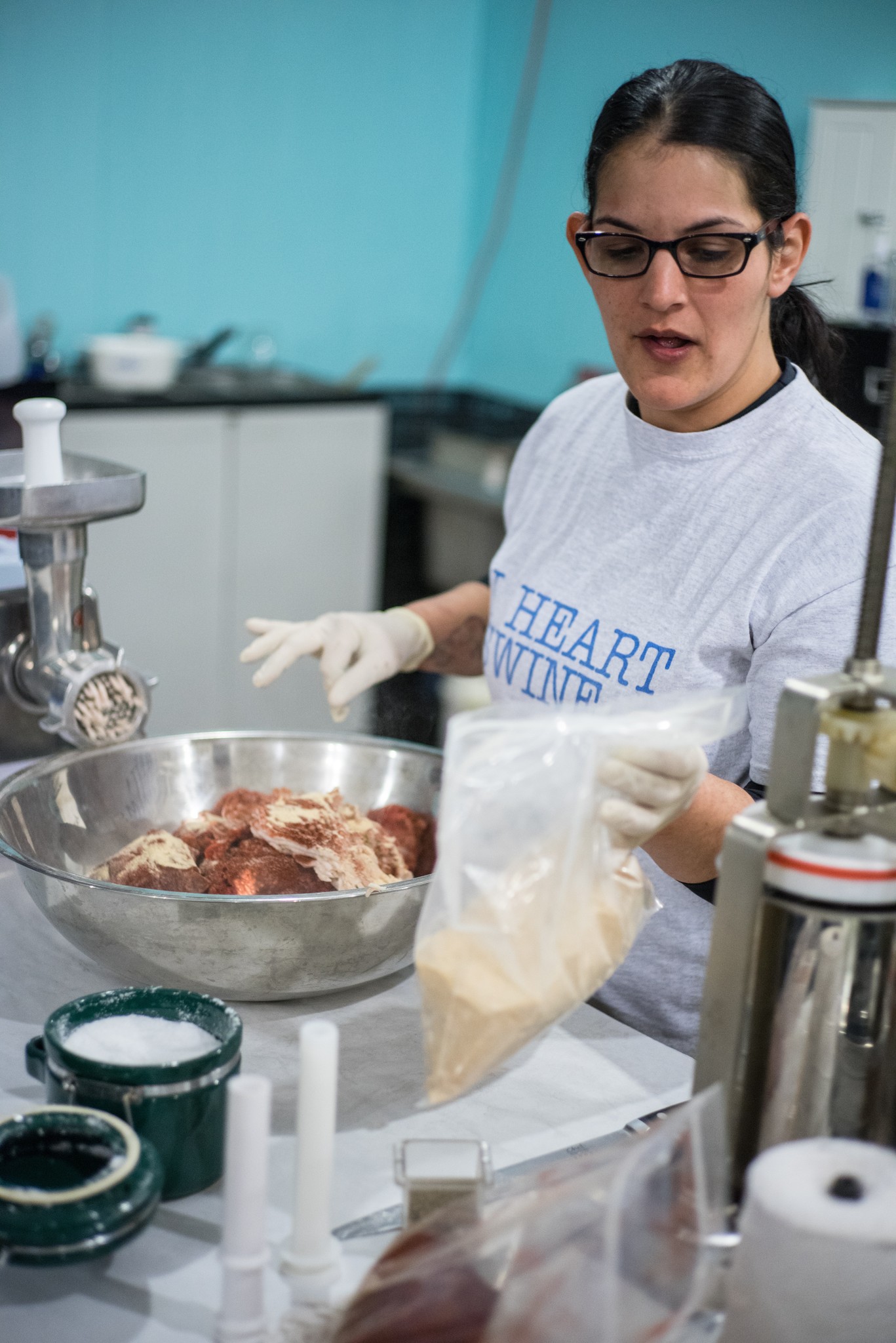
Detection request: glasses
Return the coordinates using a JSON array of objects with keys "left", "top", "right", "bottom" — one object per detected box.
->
[{"left": 575, "top": 211, "right": 778, "bottom": 279}]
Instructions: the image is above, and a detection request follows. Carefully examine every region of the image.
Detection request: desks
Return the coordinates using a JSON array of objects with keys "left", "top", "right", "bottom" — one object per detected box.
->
[{"left": 5, "top": 757, "right": 730, "bottom": 1343}]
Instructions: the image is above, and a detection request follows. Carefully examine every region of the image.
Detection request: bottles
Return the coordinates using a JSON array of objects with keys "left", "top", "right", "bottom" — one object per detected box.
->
[{"left": 860, "top": 208, "right": 896, "bottom": 325}]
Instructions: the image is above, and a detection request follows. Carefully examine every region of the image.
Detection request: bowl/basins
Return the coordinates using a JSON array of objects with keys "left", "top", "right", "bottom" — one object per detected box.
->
[
  {"left": 0, "top": 728, "right": 441, "bottom": 1001},
  {"left": 22, "top": 983, "right": 244, "bottom": 1201},
  {"left": 91, "top": 335, "right": 181, "bottom": 390}
]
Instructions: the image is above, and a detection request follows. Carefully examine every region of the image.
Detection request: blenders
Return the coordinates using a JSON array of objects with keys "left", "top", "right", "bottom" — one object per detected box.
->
[{"left": 692, "top": 663, "right": 894, "bottom": 1232}]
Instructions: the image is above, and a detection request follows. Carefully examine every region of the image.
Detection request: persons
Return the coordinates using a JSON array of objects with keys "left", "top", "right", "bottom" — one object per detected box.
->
[{"left": 233, "top": 57, "right": 896, "bottom": 1083}]
